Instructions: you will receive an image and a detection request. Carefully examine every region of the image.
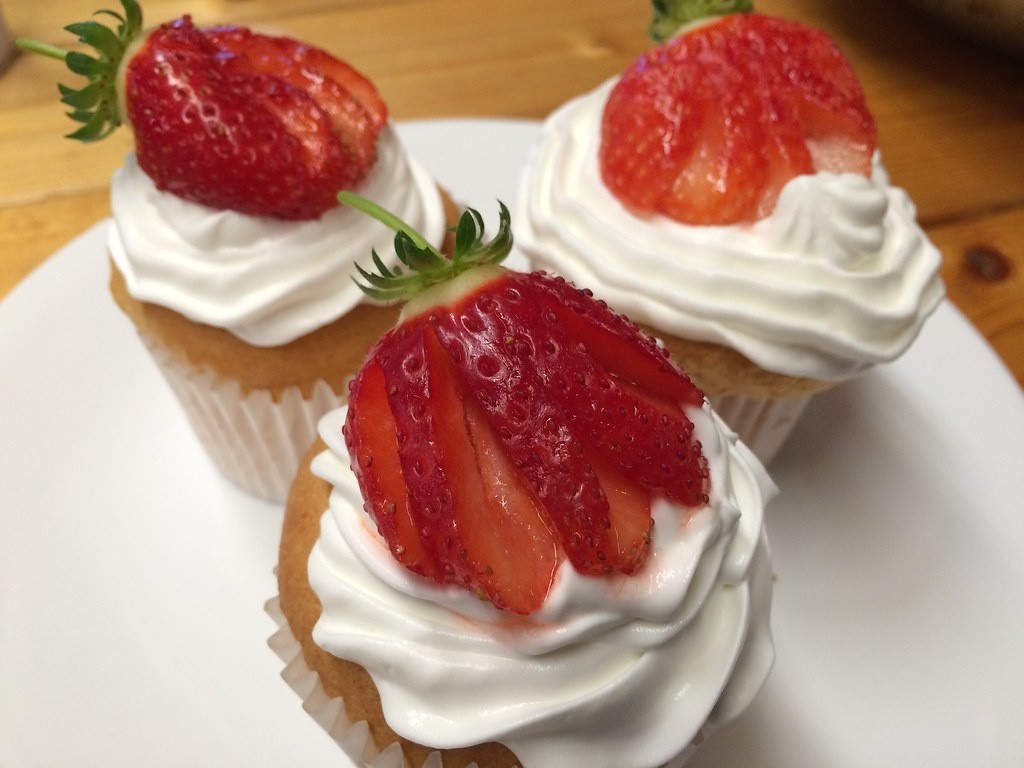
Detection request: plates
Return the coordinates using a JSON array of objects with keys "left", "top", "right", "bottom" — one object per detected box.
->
[{"left": 0, "top": 118, "right": 1024, "bottom": 768}]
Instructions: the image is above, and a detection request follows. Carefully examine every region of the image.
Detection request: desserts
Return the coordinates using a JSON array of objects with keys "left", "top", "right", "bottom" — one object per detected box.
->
[
  {"left": 516, "top": 0, "right": 944, "bottom": 469},
  {"left": 13, "top": 0, "right": 460, "bottom": 499},
  {"left": 276, "top": 188, "right": 778, "bottom": 768}
]
